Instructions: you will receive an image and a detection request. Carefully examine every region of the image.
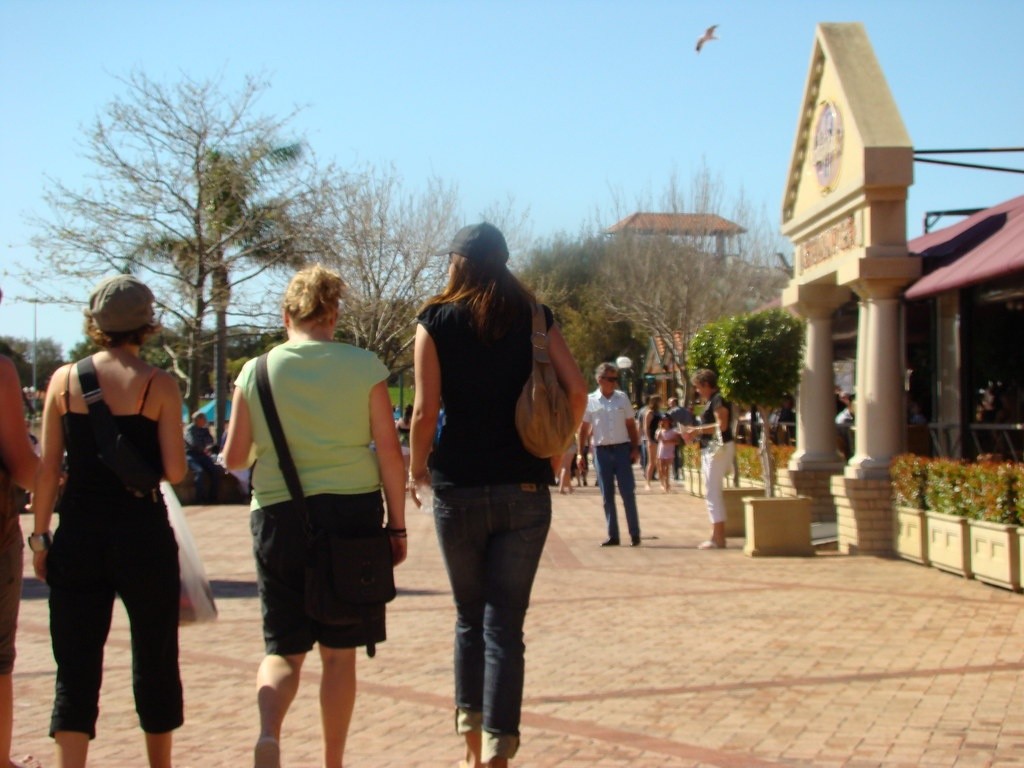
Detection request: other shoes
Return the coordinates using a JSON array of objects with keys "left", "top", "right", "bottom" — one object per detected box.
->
[{"left": 254, "top": 737, "right": 281, "bottom": 768}]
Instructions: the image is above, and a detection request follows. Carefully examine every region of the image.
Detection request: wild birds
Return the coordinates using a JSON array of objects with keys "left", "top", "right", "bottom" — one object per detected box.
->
[{"left": 695, "top": 23, "right": 718, "bottom": 52}]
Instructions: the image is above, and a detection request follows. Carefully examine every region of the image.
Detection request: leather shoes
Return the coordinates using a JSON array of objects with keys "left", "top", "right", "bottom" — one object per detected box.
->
[
  {"left": 600, "top": 537, "right": 619, "bottom": 546},
  {"left": 630, "top": 533, "right": 641, "bottom": 546}
]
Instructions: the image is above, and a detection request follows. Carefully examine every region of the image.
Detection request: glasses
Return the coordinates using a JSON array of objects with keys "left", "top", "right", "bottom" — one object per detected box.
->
[{"left": 603, "top": 376, "right": 618, "bottom": 383}]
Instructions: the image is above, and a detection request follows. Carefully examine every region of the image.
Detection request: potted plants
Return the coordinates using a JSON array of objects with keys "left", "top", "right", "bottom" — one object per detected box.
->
[{"left": 682, "top": 309, "right": 1024, "bottom": 592}]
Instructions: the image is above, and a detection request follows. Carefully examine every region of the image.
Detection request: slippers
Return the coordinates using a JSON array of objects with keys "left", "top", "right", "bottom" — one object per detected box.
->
[{"left": 698, "top": 541, "right": 727, "bottom": 550}]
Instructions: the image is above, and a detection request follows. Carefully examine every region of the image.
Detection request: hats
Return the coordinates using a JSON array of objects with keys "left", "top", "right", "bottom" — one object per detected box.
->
[
  {"left": 90, "top": 274, "right": 156, "bottom": 332},
  {"left": 434, "top": 223, "right": 509, "bottom": 268}
]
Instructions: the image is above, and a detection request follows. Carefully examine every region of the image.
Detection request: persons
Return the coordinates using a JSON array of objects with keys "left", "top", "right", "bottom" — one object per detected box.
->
[
  {"left": 221, "top": 263, "right": 409, "bottom": 768},
  {"left": 552, "top": 361, "right": 641, "bottom": 547},
  {"left": 27, "top": 273, "right": 190, "bottom": 768},
  {"left": 0, "top": 287, "right": 67, "bottom": 768},
  {"left": 901, "top": 336, "right": 1024, "bottom": 460},
  {"left": 387, "top": 402, "right": 446, "bottom": 448},
  {"left": 408, "top": 220, "right": 589, "bottom": 768},
  {"left": 684, "top": 369, "right": 737, "bottom": 551},
  {"left": 635, "top": 390, "right": 695, "bottom": 494},
  {"left": 744, "top": 394, "right": 797, "bottom": 445},
  {"left": 181, "top": 410, "right": 228, "bottom": 461},
  {"left": 832, "top": 380, "right": 855, "bottom": 460}
]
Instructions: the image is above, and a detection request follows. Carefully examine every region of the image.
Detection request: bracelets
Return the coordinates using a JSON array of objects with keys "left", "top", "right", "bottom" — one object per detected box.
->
[
  {"left": 29, "top": 530, "right": 52, "bottom": 553},
  {"left": 386, "top": 523, "right": 407, "bottom": 538},
  {"left": 410, "top": 468, "right": 430, "bottom": 491},
  {"left": 698, "top": 424, "right": 703, "bottom": 435}
]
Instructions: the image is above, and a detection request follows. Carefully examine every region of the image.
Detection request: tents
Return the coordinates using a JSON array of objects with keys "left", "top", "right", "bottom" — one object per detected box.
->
[{"left": 192, "top": 398, "right": 231, "bottom": 423}]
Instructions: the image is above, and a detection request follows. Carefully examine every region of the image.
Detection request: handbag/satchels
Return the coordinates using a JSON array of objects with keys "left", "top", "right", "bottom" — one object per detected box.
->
[
  {"left": 709, "top": 423, "right": 724, "bottom": 459},
  {"left": 159, "top": 480, "right": 219, "bottom": 627},
  {"left": 515, "top": 297, "right": 575, "bottom": 459}
]
